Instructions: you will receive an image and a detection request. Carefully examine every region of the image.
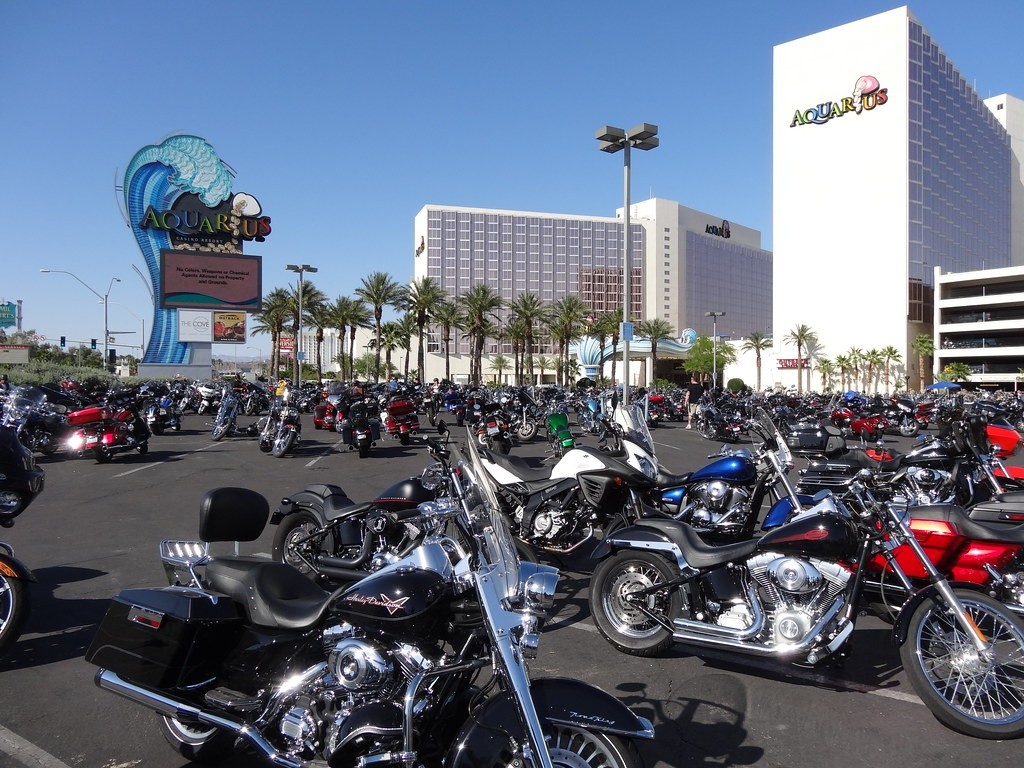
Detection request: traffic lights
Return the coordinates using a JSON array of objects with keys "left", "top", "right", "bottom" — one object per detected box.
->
[
  {"left": 61, "top": 336, "right": 65, "bottom": 347},
  {"left": 91, "top": 339, "right": 97, "bottom": 349}
]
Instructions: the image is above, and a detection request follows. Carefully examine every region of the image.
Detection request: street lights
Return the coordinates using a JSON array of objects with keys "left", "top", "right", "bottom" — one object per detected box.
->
[
  {"left": 595, "top": 123, "right": 660, "bottom": 405},
  {"left": 40, "top": 269, "right": 121, "bottom": 370},
  {"left": 246, "top": 347, "right": 262, "bottom": 375},
  {"left": 98, "top": 301, "right": 145, "bottom": 356},
  {"left": 286, "top": 264, "right": 318, "bottom": 389},
  {"left": 705, "top": 311, "right": 726, "bottom": 390}
]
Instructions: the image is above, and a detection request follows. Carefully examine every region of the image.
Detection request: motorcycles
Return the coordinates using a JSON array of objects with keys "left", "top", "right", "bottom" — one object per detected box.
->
[
  {"left": 296, "top": 381, "right": 462, "bottom": 459},
  {"left": 85, "top": 424, "right": 656, "bottom": 768},
  {"left": 589, "top": 438, "right": 1023, "bottom": 739},
  {"left": 0, "top": 373, "right": 303, "bottom": 650},
  {"left": 269, "top": 418, "right": 545, "bottom": 642},
  {"left": 460, "top": 385, "right": 1023, "bottom": 620}
]
